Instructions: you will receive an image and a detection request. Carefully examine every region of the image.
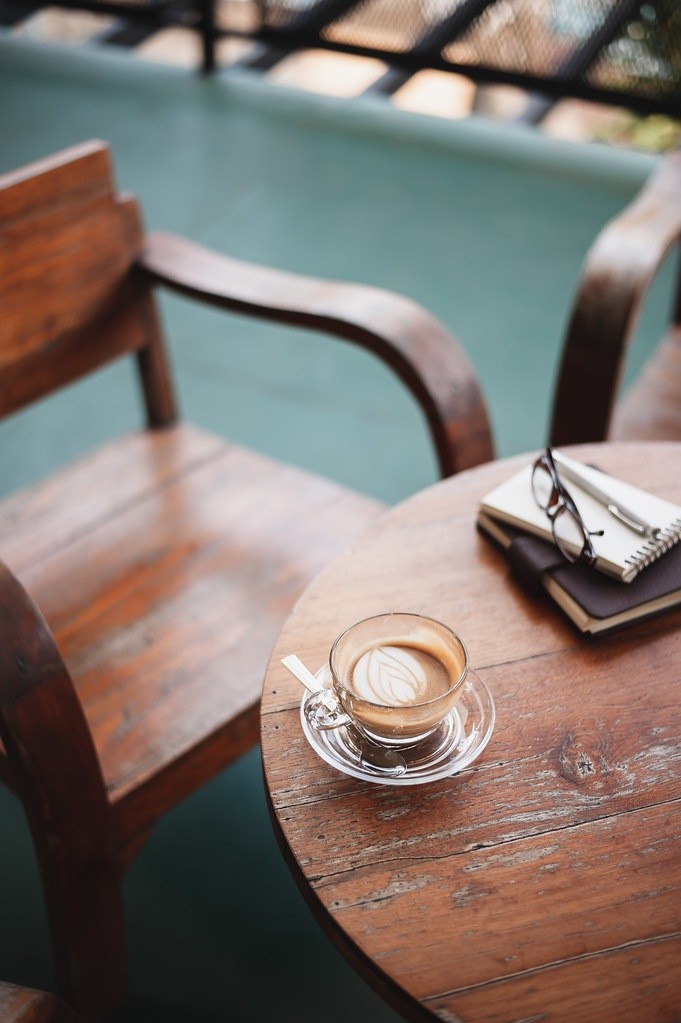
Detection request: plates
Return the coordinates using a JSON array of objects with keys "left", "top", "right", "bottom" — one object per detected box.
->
[{"left": 299, "top": 663, "right": 495, "bottom": 786}]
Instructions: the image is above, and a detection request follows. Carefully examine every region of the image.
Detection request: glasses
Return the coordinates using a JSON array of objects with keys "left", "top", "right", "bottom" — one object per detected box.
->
[{"left": 531, "top": 448, "right": 603, "bottom": 569}]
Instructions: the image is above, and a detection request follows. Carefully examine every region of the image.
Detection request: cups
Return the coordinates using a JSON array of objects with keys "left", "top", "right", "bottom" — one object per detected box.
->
[{"left": 303, "top": 613, "right": 468, "bottom": 751}]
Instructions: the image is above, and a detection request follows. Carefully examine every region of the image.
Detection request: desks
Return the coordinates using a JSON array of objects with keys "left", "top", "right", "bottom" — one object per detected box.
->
[{"left": 259, "top": 439, "right": 681, "bottom": 1023}]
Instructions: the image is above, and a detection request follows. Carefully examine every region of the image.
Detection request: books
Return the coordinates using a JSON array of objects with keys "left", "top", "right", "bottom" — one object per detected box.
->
[{"left": 477, "top": 447, "right": 681, "bottom": 640}]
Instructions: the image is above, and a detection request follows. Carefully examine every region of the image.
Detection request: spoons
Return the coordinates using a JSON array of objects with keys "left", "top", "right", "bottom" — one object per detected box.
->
[{"left": 280, "top": 653, "right": 407, "bottom": 776}]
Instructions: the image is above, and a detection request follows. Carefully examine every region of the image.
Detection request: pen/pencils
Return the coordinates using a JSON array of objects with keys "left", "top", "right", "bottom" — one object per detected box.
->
[{"left": 537, "top": 453, "right": 661, "bottom": 542}]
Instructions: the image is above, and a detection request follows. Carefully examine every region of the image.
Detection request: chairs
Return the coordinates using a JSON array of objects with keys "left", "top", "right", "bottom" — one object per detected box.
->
[
  {"left": 0, "top": 140, "right": 496, "bottom": 1023},
  {"left": 548, "top": 146, "right": 681, "bottom": 449}
]
